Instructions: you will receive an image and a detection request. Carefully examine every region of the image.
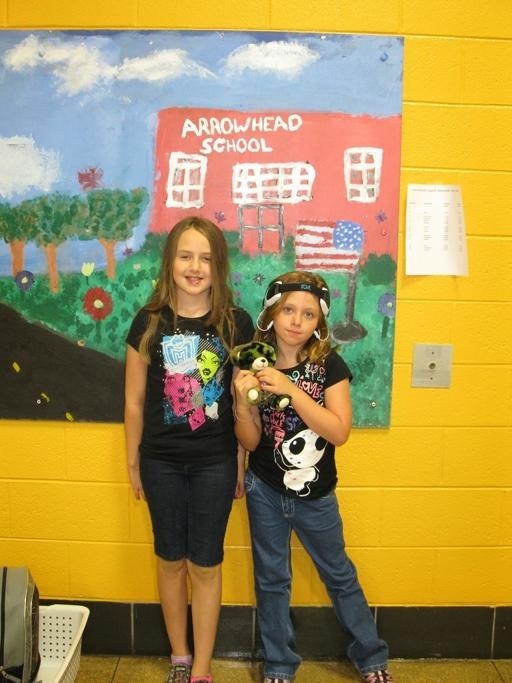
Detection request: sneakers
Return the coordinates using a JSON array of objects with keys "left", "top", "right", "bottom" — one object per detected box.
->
[
  {"left": 166, "top": 658, "right": 213, "bottom": 683},
  {"left": 262, "top": 675, "right": 292, "bottom": 683},
  {"left": 360, "top": 666, "right": 394, "bottom": 683}
]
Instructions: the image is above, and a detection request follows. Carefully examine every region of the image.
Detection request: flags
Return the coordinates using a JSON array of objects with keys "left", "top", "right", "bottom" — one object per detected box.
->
[{"left": 292, "top": 220, "right": 368, "bottom": 273}]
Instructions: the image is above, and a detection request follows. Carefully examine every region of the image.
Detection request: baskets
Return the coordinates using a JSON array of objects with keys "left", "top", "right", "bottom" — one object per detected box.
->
[{"left": 29, "top": 600, "right": 91, "bottom": 683}]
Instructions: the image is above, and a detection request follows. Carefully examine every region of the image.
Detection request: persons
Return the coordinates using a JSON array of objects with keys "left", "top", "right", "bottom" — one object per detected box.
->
[
  {"left": 123, "top": 214, "right": 252, "bottom": 682},
  {"left": 231, "top": 267, "right": 395, "bottom": 683}
]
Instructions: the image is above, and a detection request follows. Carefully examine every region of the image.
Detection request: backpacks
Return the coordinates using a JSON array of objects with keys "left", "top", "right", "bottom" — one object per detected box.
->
[{"left": 0, "top": 565, "right": 42, "bottom": 683}]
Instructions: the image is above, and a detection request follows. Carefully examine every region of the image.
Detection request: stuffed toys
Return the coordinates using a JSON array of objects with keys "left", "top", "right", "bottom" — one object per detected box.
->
[{"left": 230, "top": 341, "right": 292, "bottom": 409}]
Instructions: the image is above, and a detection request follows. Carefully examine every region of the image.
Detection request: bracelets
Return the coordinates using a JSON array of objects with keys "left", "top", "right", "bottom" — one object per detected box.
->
[{"left": 233, "top": 406, "right": 254, "bottom": 422}]
